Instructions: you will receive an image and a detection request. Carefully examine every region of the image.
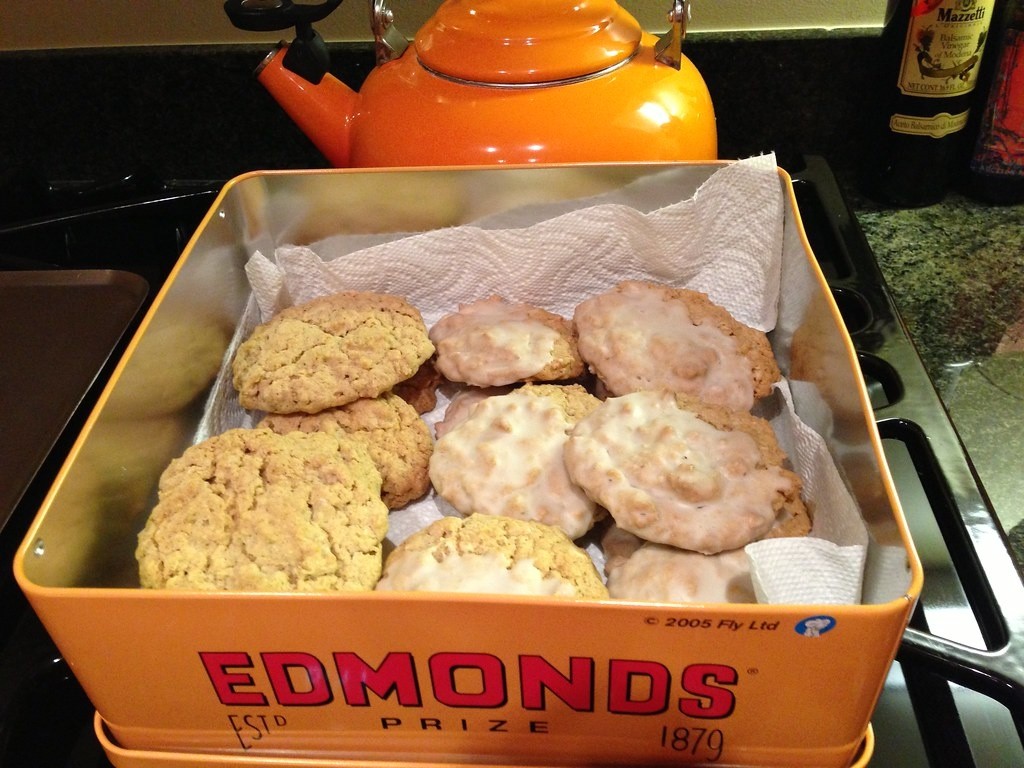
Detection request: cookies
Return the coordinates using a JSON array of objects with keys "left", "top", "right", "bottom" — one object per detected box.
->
[{"left": 132, "top": 279, "right": 812, "bottom": 607}]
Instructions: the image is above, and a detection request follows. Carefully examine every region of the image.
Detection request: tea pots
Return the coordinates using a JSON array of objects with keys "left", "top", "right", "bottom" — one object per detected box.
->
[{"left": 221, "top": 0, "right": 719, "bottom": 169}]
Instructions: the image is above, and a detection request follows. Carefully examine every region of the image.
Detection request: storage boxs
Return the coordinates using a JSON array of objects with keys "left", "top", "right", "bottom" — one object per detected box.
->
[{"left": 13, "top": 157, "right": 924, "bottom": 768}]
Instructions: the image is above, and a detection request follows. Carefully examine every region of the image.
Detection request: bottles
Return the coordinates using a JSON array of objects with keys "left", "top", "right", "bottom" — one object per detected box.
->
[
  {"left": 958, "top": 0, "right": 1024, "bottom": 206},
  {"left": 852, "top": 0, "right": 999, "bottom": 207}
]
